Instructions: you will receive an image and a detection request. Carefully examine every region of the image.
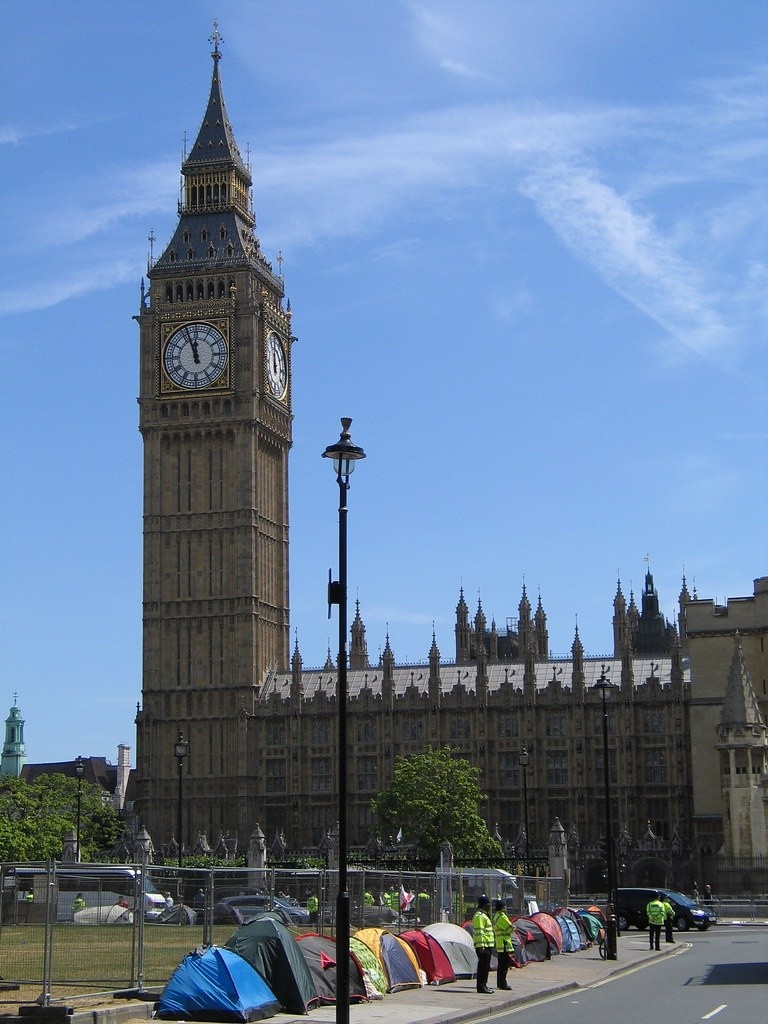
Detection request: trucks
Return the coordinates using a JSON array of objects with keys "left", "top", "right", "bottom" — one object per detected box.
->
[{"left": 435, "top": 867, "right": 535, "bottom": 915}]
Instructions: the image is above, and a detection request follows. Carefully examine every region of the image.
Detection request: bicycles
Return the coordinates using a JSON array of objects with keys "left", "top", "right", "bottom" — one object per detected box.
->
[{"left": 598, "top": 926, "right": 609, "bottom": 961}]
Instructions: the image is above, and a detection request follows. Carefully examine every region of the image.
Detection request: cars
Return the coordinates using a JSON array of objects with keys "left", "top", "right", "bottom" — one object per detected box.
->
[{"left": 196, "top": 884, "right": 313, "bottom": 926}]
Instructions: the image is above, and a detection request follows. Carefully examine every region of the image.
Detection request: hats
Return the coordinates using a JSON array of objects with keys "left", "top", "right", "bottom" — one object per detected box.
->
[
  {"left": 496, "top": 900, "right": 506, "bottom": 911},
  {"left": 478, "top": 894, "right": 490, "bottom": 909}
]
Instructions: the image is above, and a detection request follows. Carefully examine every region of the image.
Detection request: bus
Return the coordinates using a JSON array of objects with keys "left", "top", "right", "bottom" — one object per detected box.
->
[{"left": 13, "top": 866, "right": 166, "bottom": 924}]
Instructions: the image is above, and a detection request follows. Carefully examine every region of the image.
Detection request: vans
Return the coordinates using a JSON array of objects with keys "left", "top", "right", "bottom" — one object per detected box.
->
[{"left": 614, "top": 887, "right": 718, "bottom": 933}]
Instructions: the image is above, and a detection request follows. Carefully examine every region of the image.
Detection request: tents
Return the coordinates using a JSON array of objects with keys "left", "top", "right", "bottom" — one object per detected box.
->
[
  {"left": 74, "top": 905, "right": 133, "bottom": 925},
  {"left": 156, "top": 907, "right": 606, "bottom": 1024}
]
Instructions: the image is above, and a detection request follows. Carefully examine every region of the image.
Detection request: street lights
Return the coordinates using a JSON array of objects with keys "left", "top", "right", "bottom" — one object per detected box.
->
[
  {"left": 72, "top": 756, "right": 85, "bottom": 863},
  {"left": 592, "top": 675, "right": 618, "bottom": 960},
  {"left": 173, "top": 729, "right": 189, "bottom": 875},
  {"left": 517, "top": 744, "right": 531, "bottom": 876},
  {"left": 321, "top": 416, "right": 368, "bottom": 1023}
]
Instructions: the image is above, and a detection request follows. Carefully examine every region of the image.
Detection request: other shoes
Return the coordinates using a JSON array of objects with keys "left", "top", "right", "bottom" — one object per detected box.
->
[
  {"left": 497, "top": 983, "right": 512, "bottom": 990},
  {"left": 656, "top": 948, "right": 661, "bottom": 950},
  {"left": 477, "top": 987, "right": 494, "bottom": 993}
]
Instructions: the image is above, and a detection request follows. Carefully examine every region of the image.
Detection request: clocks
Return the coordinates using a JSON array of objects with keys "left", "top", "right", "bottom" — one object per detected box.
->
[
  {"left": 265, "top": 332, "right": 289, "bottom": 399},
  {"left": 162, "top": 321, "right": 229, "bottom": 389}
]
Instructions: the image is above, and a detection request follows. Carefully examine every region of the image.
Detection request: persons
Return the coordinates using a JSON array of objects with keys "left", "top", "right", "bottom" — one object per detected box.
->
[
  {"left": 165, "top": 892, "right": 173, "bottom": 909},
  {"left": 646, "top": 893, "right": 665, "bottom": 951},
  {"left": 383, "top": 886, "right": 430, "bottom": 912},
  {"left": 307, "top": 890, "right": 319, "bottom": 924},
  {"left": 691, "top": 885, "right": 698, "bottom": 899},
  {"left": 704, "top": 885, "right": 714, "bottom": 908},
  {"left": 27, "top": 887, "right": 33, "bottom": 902},
  {"left": 492, "top": 900, "right": 515, "bottom": 990},
  {"left": 116, "top": 896, "right": 128, "bottom": 908},
  {"left": 281, "top": 886, "right": 290, "bottom": 898},
  {"left": 193, "top": 889, "right": 205, "bottom": 908},
  {"left": 364, "top": 888, "right": 375, "bottom": 906},
  {"left": 72, "top": 893, "right": 85, "bottom": 912},
  {"left": 662, "top": 895, "right": 676, "bottom": 943},
  {"left": 473, "top": 894, "right": 495, "bottom": 994}
]
